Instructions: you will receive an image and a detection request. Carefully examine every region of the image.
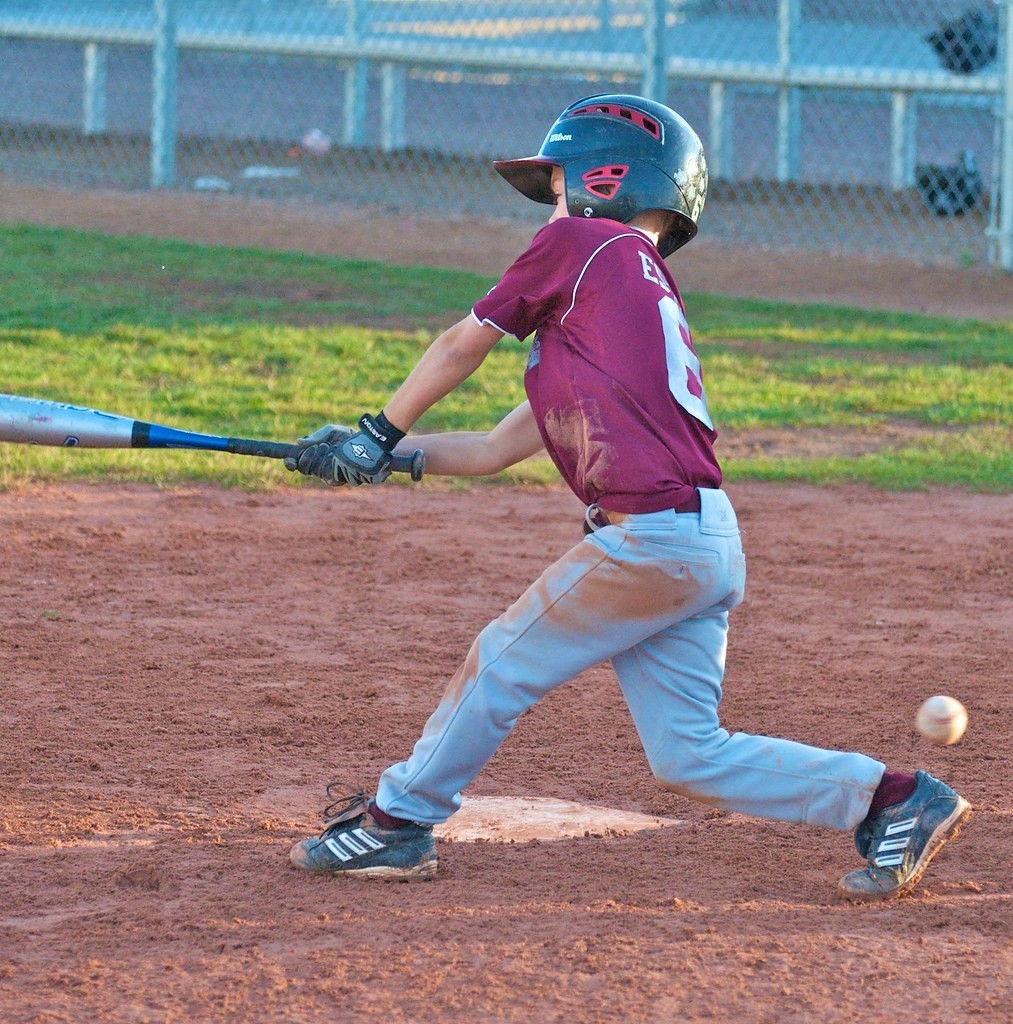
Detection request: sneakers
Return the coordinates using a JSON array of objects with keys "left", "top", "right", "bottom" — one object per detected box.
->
[
  {"left": 288, "top": 782, "right": 438, "bottom": 881},
  {"left": 838, "top": 770, "right": 971, "bottom": 900}
]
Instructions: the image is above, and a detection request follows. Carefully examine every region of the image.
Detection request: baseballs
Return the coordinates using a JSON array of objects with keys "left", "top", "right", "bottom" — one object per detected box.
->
[{"left": 916, "top": 695, "right": 969, "bottom": 746}]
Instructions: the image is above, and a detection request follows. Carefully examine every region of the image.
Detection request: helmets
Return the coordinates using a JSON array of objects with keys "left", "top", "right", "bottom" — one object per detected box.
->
[{"left": 493, "top": 93, "right": 707, "bottom": 259}]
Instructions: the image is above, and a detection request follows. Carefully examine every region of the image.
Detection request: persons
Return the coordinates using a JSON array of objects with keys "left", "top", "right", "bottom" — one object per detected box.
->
[{"left": 279, "top": 93, "right": 971, "bottom": 903}]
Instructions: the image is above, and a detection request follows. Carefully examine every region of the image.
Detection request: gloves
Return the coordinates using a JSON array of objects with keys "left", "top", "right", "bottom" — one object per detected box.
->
[
  {"left": 331, "top": 411, "right": 409, "bottom": 485},
  {"left": 285, "top": 423, "right": 358, "bottom": 485}
]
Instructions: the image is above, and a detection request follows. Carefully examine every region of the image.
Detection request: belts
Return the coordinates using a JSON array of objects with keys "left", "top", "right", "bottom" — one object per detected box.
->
[{"left": 584, "top": 489, "right": 704, "bottom": 533}]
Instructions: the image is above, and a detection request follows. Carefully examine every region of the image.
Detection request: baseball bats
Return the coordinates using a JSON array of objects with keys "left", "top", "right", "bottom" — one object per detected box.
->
[{"left": 0, "top": 388, "right": 429, "bottom": 483}]
